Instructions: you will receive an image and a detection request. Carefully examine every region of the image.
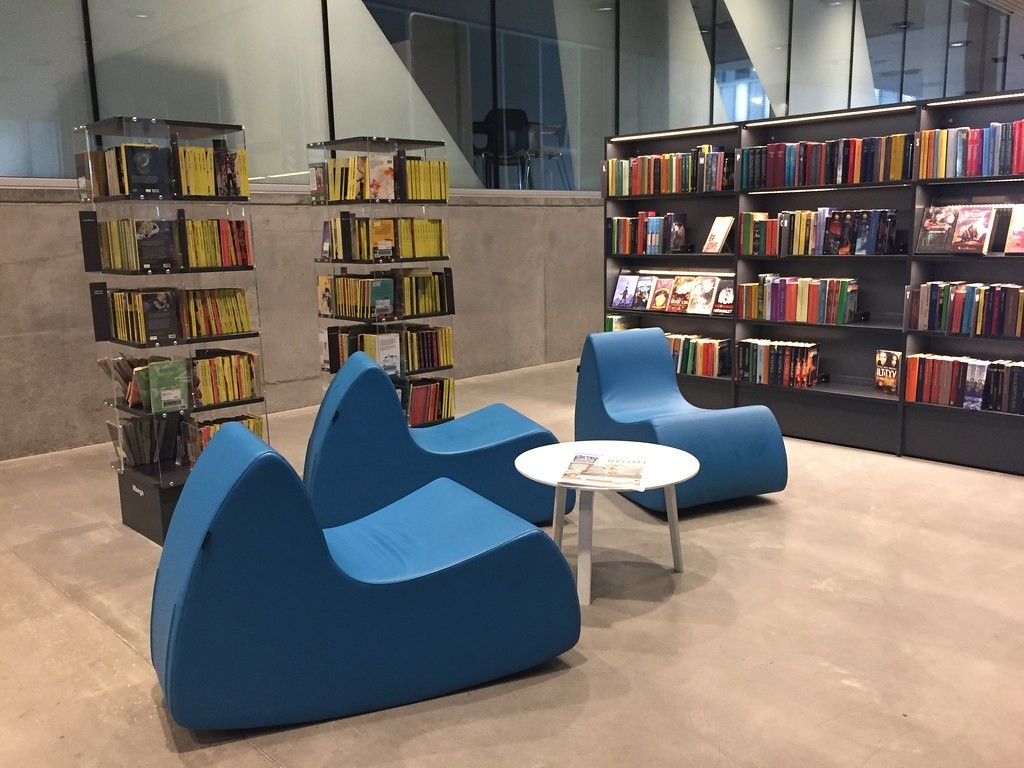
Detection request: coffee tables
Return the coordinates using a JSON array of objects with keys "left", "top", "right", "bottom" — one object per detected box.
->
[{"left": 514, "top": 439, "right": 701, "bottom": 606}]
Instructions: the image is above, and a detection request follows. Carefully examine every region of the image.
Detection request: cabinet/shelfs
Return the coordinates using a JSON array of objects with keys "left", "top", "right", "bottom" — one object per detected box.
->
[
  {"left": 76, "top": 114, "right": 270, "bottom": 550},
  {"left": 604, "top": 90, "right": 1024, "bottom": 481},
  {"left": 306, "top": 135, "right": 458, "bottom": 427}
]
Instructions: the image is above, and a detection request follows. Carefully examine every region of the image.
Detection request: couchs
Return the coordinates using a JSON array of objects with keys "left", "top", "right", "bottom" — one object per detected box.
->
[
  {"left": 574, "top": 327, "right": 787, "bottom": 514},
  {"left": 151, "top": 420, "right": 581, "bottom": 733},
  {"left": 302, "top": 351, "right": 576, "bottom": 538}
]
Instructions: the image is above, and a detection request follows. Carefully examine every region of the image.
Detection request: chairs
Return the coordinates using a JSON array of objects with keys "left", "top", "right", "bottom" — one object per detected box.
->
[
  {"left": 525, "top": 118, "right": 575, "bottom": 191},
  {"left": 479, "top": 107, "right": 533, "bottom": 189}
]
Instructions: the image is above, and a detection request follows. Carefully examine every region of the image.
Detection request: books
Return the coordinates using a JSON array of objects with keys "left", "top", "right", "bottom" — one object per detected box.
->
[
  {"left": 75, "top": 141, "right": 268, "bottom": 477},
  {"left": 603, "top": 120, "right": 1023, "bottom": 414},
  {"left": 309, "top": 157, "right": 457, "bottom": 429}
]
